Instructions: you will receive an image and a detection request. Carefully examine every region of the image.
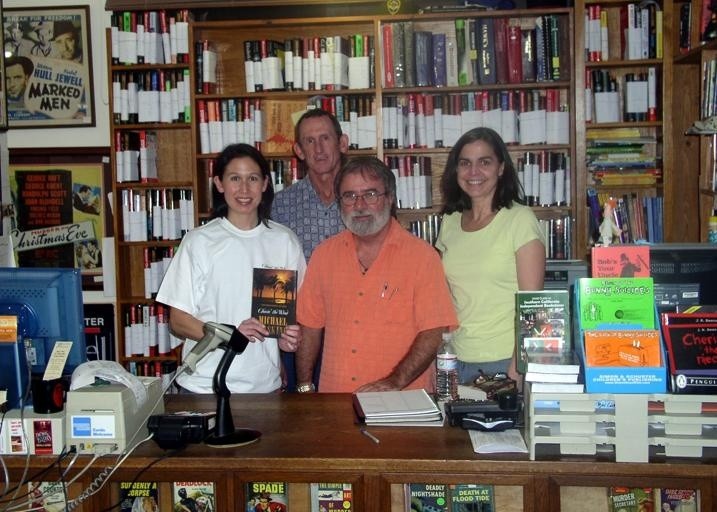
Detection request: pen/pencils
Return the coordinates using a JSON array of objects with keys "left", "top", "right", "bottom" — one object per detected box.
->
[
  {"left": 361, "top": 427, "right": 380, "bottom": 444},
  {"left": 380, "top": 282, "right": 399, "bottom": 302}
]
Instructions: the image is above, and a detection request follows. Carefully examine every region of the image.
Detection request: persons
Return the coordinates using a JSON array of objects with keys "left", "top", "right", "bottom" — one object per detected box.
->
[
  {"left": 85, "top": 240, "right": 102, "bottom": 269},
  {"left": 268, "top": 109, "right": 350, "bottom": 393},
  {"left": 255, "top": 490, "right": 286, "bottom": 512},
  {"left": 80, "top": 194, "right": 100, "bottom": 215},
  {"left": 30, "top": 19, "right": 61, "bottom": 59},
  {"left": 76, "top": 243, "right": 99, "bottom": 269},
  {"left": 48, "top": 18, "right": 85, "bottom": 119},
  {"left": 296, "top": 155, "right": 461, "bottom": 392},
  {"left": 29, "top": 481, "right": 50, "bottom": 512},
  {"left": 318, "top": 491, "right": 341, "bottom": 500},
  {"left": 4, "top": 21, "right": 35, "bottom": 57},
  {"left": 142, "top": 497, "right": 157, "bottom": 512},
  {"left": 177, "top": 488, "right": 204, "bottom": 512},
  {"left": 73, "top": 185, "right": 92, "bottom": 210},
  {"left": 4, "top": 55, "right": 34, "bottom": 101},
  {"left": 431, "top": 127, "right": 549, "bottom": 393},
  {"left": 155, "top": 143, "right": 307, "bottom": 395},
  {"left": 248, "top": 497, "right": 256, "bottom": 512}
]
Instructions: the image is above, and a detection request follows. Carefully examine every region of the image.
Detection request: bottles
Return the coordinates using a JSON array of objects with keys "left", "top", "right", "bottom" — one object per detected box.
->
[{"left": 436, "top": 332, "right": 457, "bottom": 400}]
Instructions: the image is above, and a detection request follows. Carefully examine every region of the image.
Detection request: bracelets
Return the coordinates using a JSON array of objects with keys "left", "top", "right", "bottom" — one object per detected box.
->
[{"left": 295, "top": 384, "right": 316, "bottom": 393}]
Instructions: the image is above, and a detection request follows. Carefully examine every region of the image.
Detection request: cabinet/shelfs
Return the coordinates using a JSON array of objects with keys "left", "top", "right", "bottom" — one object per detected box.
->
[
  {"left": 377, "top": 8, "right": 576, "bottom": 262},
  {"left": 189, "top": 16, "right": 377, "bottom": 227},
  {"left": 574, "top": 0, "right": 671, "bottom": 259},
  {"left": 104, "top": 0, "right": 197, "bottom": 395},
  {"left": 670, "top": 0, "right": 717, "bottom": 244}
]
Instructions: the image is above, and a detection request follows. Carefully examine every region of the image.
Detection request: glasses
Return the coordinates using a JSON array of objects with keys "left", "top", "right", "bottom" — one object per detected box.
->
[
  {"left": 337, "top": 188, "right": 388, "bottom": 207},
  {"left": 473, "top": 369, "right": 510, "bottom": 387}
]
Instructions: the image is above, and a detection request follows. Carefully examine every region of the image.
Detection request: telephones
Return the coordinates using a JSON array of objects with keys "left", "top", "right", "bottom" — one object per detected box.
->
[{"left": 444, "top": 391, "right": 521, "bottom": 432}]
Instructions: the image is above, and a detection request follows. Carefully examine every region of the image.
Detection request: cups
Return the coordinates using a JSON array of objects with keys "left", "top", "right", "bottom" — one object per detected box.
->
[{"left": 32, "top": 374, "right": 67, "bottom": 413}]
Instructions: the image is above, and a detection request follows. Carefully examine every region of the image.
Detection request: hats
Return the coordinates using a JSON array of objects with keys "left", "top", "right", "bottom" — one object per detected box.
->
[
  {"left": 48, "top": 19, "right": 81, "bottom": 42},
  {"left": 6, "top": 57, "right": 34, "bottom": 76},
  {"left": 620, "top": 254, "right": 629, "bottom": 261},
  {"left": 34, "top": 24, "right": 51, "bottom": 33},
  {"left": 257, "top": 490, "right": 273, "bottom": 501},
  {"left": 7, "top": 22, "right": 24, "bottom": 32}
]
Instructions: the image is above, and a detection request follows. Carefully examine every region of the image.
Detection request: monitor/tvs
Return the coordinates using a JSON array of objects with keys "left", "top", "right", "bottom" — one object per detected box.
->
[{"left": 0, "top": 267, "right": 87, "bottom": 410}]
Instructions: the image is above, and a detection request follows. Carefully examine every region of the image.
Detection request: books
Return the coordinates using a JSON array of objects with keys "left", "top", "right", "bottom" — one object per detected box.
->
[
  {"left": 311, "top": 482, "right": 353, "bottom": 512},
  {"left": 351, "top": 387, "right": 443, "bottom": 426},
  {"left": 193, "top": 33, "right": 378, "bottom": 260},
  {"left": 607, "top": 486, "right": 657, "bottom": 512},
  {"left": 251, "top": 268, "right": 298, "bottom": 338},
  {"left": 582, "top": 1, "right": 717, "bottom": 258},
  {"left": 660, "top": 488, "right": 701, "bottom": 512},
  {"left": 110, "top": 1, "right": 196, "bottom": 381},
  {"left": 404, "top": 483, "right": 448, "bottom": 512},
  {"left": 118, "top": 481, "right": 160, "bottom": 512},
  {"left": 28, "top": 481, "right": 68, "bottom": 512},
  {"left": 516, "top": 246, "right": 717, "bottom": 395},
  {"left": 449, "top": 484, "right": 495, "bottom": 512},
  {"left": 246, "top": 480, "right": 288, "bottom": 512},
  {"left": 380, "top": 14, "right": 572, "bottom": 259},
  {"left": 172, "top": 482, "right": 217, "bottom": 512}
]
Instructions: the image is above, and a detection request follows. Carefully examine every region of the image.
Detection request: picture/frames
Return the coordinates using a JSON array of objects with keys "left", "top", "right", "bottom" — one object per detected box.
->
[
  {"left": 1, "top": 148, "right": 111, "bottom": 291},
  {"left": 0, "top": 4, "right": 96, "bottom": 129}
]
